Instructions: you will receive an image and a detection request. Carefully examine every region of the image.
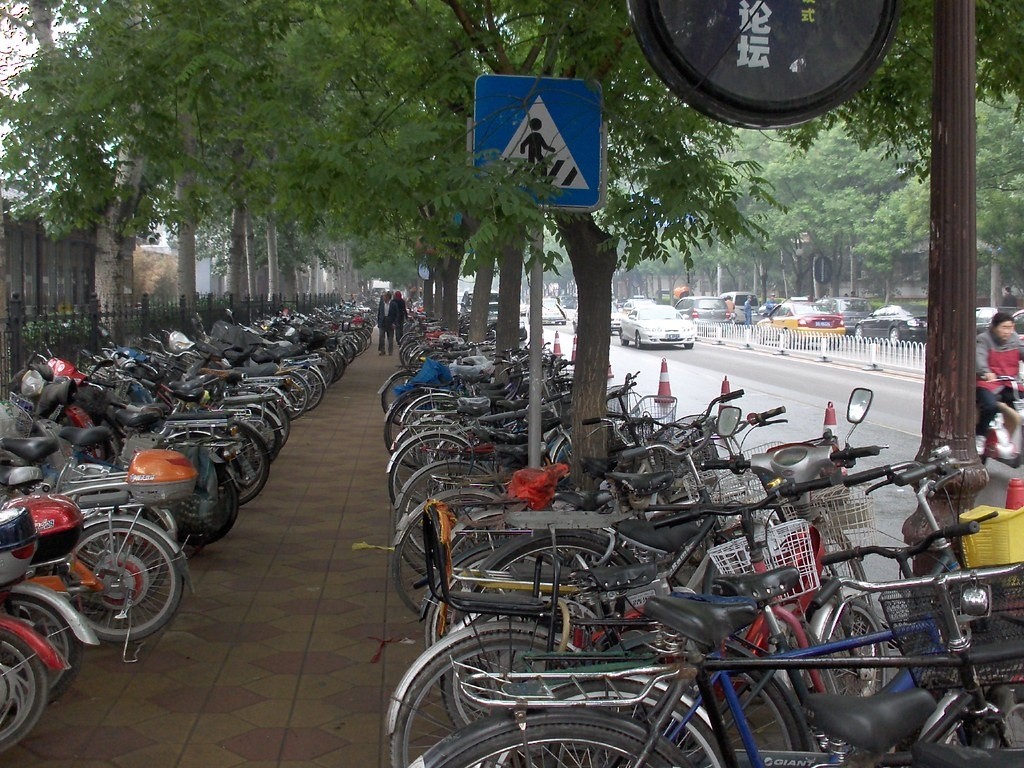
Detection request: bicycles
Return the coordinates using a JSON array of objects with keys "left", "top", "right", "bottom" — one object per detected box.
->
[{"left": 0, "top": 295, "right": 1024, "bottom": 768}]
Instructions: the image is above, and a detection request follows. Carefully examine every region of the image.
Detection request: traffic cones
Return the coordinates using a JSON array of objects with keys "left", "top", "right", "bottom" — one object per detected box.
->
[
  {"left": 553, "top": 330, "right": 564, "bottom": 357},
  {"left": 719, "top": 374, "right": 738, "bottom": 430},
  {"left": 571, "top": 335, "right": 577, "bottom": 362},
  {"left": 821, "top": 402, "right": 848, "bottom": 479},
  {"left": 1005, "top": 477, "right": 1024, "bottom": 510},
  {"left": 653, "top": 357, "right": 675, "bottom": 404}
]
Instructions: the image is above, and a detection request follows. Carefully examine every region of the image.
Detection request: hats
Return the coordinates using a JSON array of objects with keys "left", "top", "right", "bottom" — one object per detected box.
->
[{"left": 395, "top": 291, "right": 401, "bottom": 298}]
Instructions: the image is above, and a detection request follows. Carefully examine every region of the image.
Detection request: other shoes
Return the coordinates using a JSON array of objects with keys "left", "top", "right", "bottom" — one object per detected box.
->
[
  {"left": 389, "top": 352, "right": 393, "bottom": 355},
  {"left": 379, "top": 351, "right": 386, "bottom": 355}
]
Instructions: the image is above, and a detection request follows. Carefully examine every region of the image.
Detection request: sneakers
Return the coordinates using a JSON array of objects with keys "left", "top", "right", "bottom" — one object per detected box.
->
[{"left": 975, "top": 435, "right": 986, "bottom": 455}]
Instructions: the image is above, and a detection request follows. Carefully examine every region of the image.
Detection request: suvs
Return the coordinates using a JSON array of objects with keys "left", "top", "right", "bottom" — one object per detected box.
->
[{"left": 459, "top": 290, "right": 527, "bottom": 342}]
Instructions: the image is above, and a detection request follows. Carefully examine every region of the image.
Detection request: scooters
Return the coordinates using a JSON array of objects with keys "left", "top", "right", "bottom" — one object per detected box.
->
[{"left": 973, "top": 374, "right": 1024, "bottom": 472}]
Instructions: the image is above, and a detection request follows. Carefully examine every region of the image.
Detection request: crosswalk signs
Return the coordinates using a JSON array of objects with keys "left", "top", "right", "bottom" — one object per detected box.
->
[{"left": 470, "top": 74, "right": 604, "bottom": 209}]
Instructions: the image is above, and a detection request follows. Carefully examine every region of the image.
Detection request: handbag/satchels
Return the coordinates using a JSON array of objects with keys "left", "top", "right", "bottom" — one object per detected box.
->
[{"left": 173, "top": 447, "right": 218, "bottom": 525}]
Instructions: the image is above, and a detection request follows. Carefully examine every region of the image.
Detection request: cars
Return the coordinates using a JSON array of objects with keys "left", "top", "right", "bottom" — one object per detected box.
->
[
  {"left": 616, "top": 304, "right": 697, "bottom": 350},
  {"left": 540, "top": 297, "right": 567, "bottom": 326},
  {"left": 718, "top": 290, "right": 760, "bottom": 324},
  {"left": 814, "top": 297, "right": 874, "bottom": 338},
  {"left": 573, "top": 296, "right": 623, "bottom": 336},
  {"left": 975, "top": 305, "right": 1024, "bottom": 336},
  {"left": 853, "top": 303, "right": 928, "bottom": 349},
  {"left": 752, "top": 295, "right": 848, "bottom": 350},
  {"left": 673, "top": 295, "right": 734, "bottom": 337}
]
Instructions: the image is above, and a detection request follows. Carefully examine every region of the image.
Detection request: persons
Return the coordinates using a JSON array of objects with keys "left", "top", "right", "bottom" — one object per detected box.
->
[
  {"left": 390, "top": 291, "right": 408, "bottom": 345},
  {"left": 975, "top": 312, "right": 1024, "bottom": 436},
  {"left": 1002, "top": 287, "right": 1017, "bottom": 307},
  {"left": 844, "top": 291, "right": 856, "bottom": 297},
  {"left": 744, "top": 295, "right": 752, "bottom": 329},
  {"left": 387, "top": 291, "right": 391, "bottom": 299},
  {"left": 378, "top": 292, "right": 399, "bottom": 356},
  {"left": 767, "top": 293, "right": 779, "bottom": 304},
  {"left": 725, "top": 295, "right": 735, "bottom": 311}
]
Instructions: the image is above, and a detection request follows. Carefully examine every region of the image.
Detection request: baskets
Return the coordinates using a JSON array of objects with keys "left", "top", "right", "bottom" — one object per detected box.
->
[
  {"left": 682, "top": 441, "right": 784, "bottom": 533},
  {"left": 135, "top": 346, "right": 187, "bottom": 387},
  {"left": 958, "top": 505, "right": 1024, "bottom": 569},
  {"left": 707, "top": 517, "right": 820, "bottom": 605},
  {"left": 606, "top": 385, "right": 628, "bottom": 421},
  {"left": 628, "top": 395, "right": 678, "bottom": 447},
  {"left": 878, "top": 560, "right": 1024, "bottom": 690},
  {"left": 781, "top": 477, "right": 878, "bottom": 561},
  {"left": 558, "top": 369, "right": 574, "bottom": 379},
  {"left": 0, "top": 397, "right": 33, "bottom": 439},
  {"left": 650, "top": 412, "right": 720, "bottom": 477},
  {"left": 9, "top": 392, "right": 36, "bottom": 417},
  {"left": 86, "top": 364, "right": 132, "bottom": 400}
]
